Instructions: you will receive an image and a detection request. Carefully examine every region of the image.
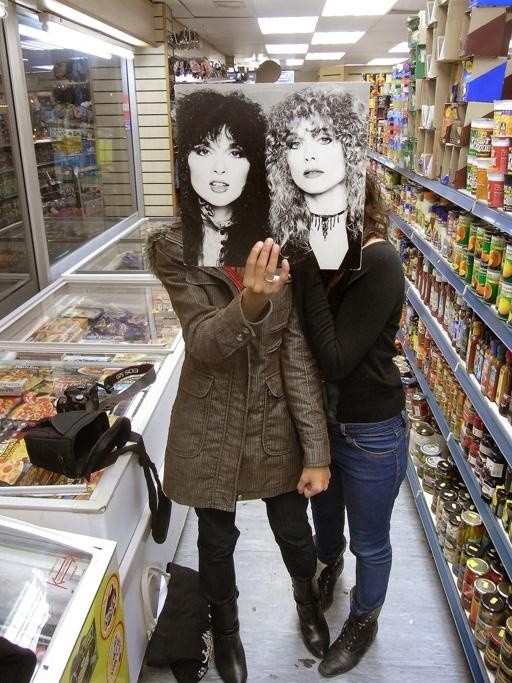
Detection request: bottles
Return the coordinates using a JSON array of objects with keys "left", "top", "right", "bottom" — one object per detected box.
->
[{"left": 390, "top": 224, "right": 510, "bottom": 458}]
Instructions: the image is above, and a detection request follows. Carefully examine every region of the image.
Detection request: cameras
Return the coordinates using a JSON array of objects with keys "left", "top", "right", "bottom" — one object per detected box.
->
[{"left": 57, "top": 384, "right": 99, "bottom": 413}]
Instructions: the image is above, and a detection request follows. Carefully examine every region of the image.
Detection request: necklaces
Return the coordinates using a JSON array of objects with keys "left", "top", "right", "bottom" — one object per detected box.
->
[
  {"left": 307, "top": 204, "right": 352, "bottom": 241},
  {"left": 201, "top": 218, "right": 234, "bottom": 235}
]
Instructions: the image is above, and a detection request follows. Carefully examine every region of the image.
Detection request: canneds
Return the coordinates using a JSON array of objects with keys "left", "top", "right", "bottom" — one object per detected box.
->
[{"left": 391, "top": 73, "right": 512, "bottom": 683}]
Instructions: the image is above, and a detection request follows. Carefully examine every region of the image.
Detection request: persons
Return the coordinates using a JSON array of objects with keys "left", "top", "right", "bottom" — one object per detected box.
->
[
  {"left": 278, "top": 169, "right": 411, "bottom": 677},
  {"left": 263, "top": 85, "right": 369, "bottom": 273},
  {"left": 145, "top": 219, "right": 331, "bottom": 682},
  {"left": 174, "top": 90, "right": 271, "bottom": 266}
]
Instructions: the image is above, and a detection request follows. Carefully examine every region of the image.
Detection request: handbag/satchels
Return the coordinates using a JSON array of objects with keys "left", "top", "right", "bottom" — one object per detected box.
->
[{"left": 146, "top": 562, "right": 216, "bottom": 682}]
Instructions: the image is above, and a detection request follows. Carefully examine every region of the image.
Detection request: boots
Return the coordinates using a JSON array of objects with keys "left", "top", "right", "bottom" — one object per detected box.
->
[
  {"left": 291, "top": 534, "right": 382, "bottom": 677},
  {"left": 207, "top": 587, "right": 247, "bottom": 683}
]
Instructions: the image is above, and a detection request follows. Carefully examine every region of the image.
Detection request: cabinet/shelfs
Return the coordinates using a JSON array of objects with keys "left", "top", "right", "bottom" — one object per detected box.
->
[
  {"left": 1, "top": 103, "right": 101, "bottom": 234},
  {"left": 361, "top": 0, "right": 512, "bottom": 682}
]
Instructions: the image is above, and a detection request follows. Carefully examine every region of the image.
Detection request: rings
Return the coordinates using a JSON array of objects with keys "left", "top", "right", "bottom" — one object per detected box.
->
[{"left": 264, "top": 276, "right": 275, "bottom": 283}]
